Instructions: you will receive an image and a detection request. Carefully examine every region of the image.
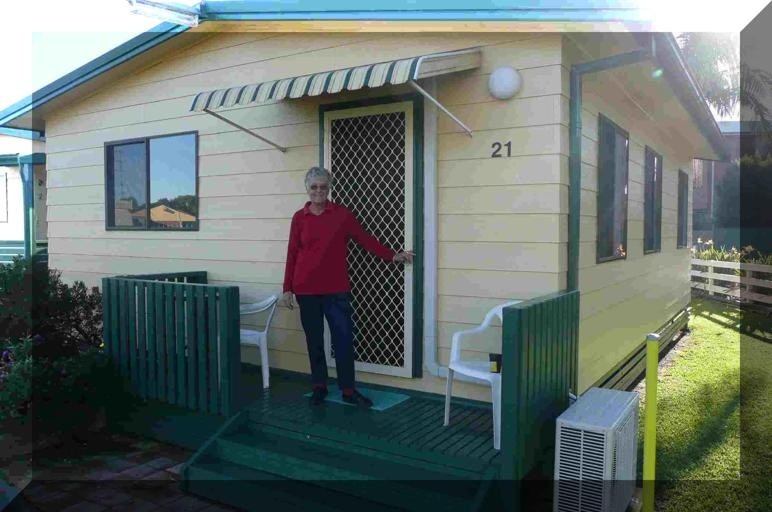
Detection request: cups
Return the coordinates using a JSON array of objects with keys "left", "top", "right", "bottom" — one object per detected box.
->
[{"left": 488, "top": 353, "right": 502, "bottom": 373}]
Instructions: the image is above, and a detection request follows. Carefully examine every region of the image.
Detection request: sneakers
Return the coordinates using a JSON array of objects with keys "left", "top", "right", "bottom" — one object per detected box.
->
[
  {"left": 343, "top": 390, "right": 373, "bottom": 407},
  {"left": 311, "top": 386, "right": 328, "bottom": 401}
]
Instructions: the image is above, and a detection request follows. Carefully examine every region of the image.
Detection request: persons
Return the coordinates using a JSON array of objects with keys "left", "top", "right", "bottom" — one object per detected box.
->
[{"left": 282, "top": 167, "right": 416, "bottom": 408}]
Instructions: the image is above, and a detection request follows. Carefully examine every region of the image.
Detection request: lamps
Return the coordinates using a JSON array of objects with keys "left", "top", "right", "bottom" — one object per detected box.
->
[{"left": 487, "top": 66, "right": 523, "bottom": 101}]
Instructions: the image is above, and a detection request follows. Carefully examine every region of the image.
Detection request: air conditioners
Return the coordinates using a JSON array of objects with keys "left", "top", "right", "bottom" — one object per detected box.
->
[{"left": 552, "top": 387, "right": 640, "bottom": 512}]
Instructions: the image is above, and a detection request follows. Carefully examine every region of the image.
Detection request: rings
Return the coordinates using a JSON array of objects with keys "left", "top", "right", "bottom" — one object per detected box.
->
[{"left": 406, "top": 251, "right": 409, "bottom": 254}]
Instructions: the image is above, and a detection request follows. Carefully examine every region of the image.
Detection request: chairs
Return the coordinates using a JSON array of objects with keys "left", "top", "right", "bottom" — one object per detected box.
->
[
  {"left": 216, "top": 295, "right": 279, "bottom": 388},
  {"left": 442, "top": 300, "right": 524, "bottom": 450}
]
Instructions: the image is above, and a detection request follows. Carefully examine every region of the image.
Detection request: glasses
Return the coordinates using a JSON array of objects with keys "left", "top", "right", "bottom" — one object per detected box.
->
[{"left": 311, "top": 186, "right": 327, "bottom": 190}]
